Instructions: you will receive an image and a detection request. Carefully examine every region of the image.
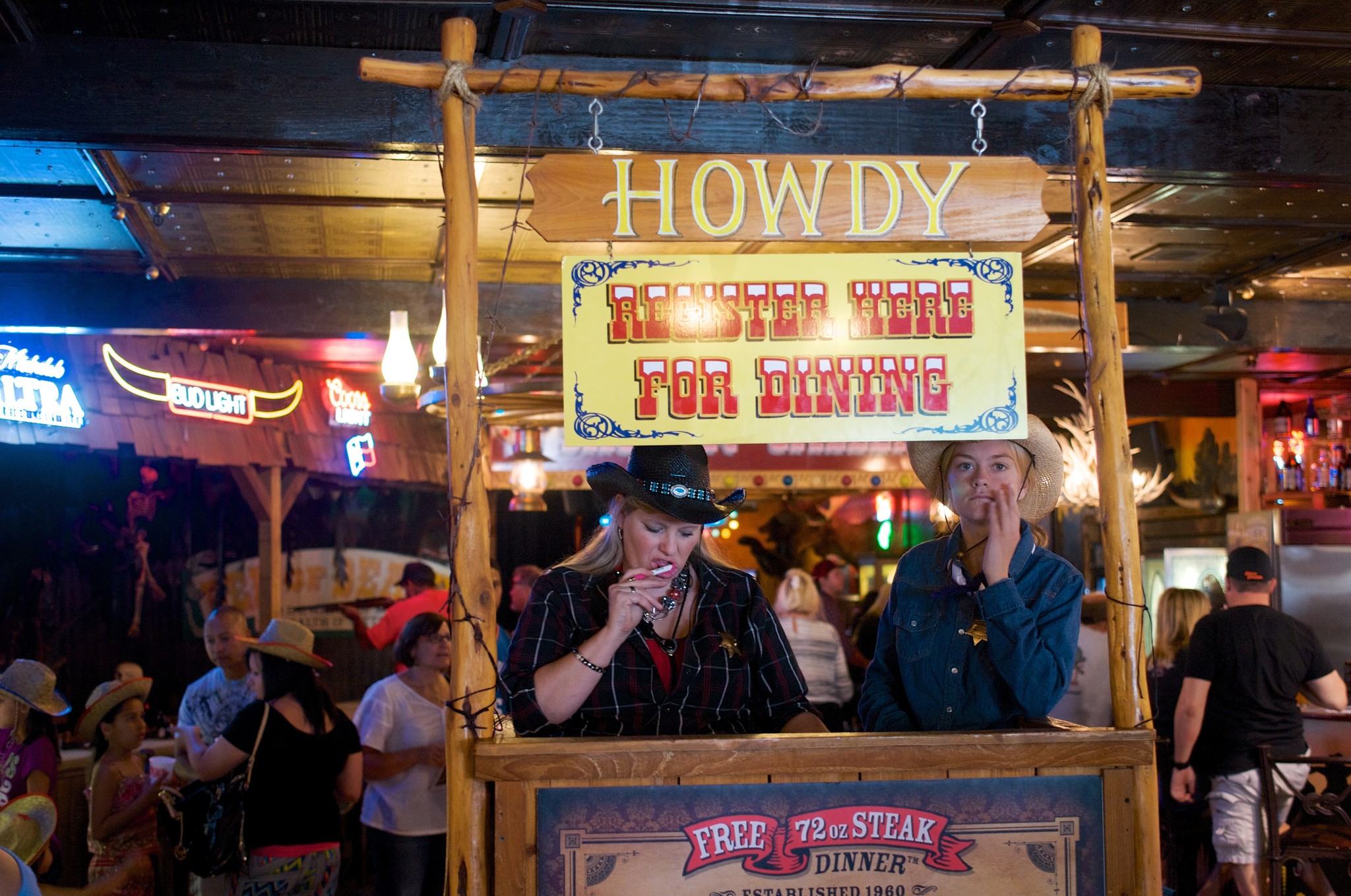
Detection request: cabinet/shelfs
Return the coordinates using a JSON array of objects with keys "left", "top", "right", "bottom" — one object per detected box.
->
[{"left": 1256, "top": 379, "right": 1351, "bottom": 512}]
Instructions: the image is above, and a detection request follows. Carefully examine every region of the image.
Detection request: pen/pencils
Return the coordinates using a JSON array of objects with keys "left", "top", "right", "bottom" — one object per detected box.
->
[{"left": 626, "top": 564, "right": 676, "bottom": 582}]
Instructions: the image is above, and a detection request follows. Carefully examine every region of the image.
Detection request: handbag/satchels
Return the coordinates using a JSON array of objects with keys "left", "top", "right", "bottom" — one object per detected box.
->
[{"left": 159, "top": 701, "right": 270, "bottom": 879}]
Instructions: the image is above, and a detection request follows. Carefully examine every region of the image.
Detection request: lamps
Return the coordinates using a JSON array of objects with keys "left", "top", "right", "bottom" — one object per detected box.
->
[
  {"left": 379, "top": 287, "right": 565, "bottom": 429},
  {"left": 502, "top": 448, "right": 556, "bottom": 511},
  {"left": 1200, "top": 289, "right": 1250, "bottom": 342}
]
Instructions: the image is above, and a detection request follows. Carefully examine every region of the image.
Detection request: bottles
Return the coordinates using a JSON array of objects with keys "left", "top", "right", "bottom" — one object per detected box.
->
[
  {"left": 1327, "top": 397, "right": 1344, "bottom": 439},
  {"left": 1313, "top": 446, "right": 1351, "bottom": 491},
  {"left": 1283, "top": 447, "right": 1304, "bottom": 491},
  {"left": 1274, "top": 401, "right": 1291, "bottom": 436},
  {"left": 1305, "top": 397, "right": 1321, "bottom": 439}
]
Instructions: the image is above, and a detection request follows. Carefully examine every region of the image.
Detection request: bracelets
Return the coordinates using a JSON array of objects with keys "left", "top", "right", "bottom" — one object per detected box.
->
[
  {"left": 1172, "top": 759, "right": 1190, "bottom": 770},
  {"left": 572, "top": 645, "right": 607, "bottom": 673}
]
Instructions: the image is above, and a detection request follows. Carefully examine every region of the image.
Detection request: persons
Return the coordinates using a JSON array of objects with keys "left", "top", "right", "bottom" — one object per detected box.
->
[
  {"left": 0, "top": 501, "right": 1351, "bottom": 896},
  {"left": 856, "top": 413, "right": 1085, "bottom": 732},
  {"left": 499, "top": 446, "right": 836, "bottom": 733}
]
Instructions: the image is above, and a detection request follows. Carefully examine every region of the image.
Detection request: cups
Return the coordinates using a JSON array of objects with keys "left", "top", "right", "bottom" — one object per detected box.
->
[{"left": 150, "top": 756, "right": 175, "bottom": 785}]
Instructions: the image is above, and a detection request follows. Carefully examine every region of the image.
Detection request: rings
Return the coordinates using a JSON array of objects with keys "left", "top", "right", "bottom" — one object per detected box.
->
[{"left": 630, "top": 587, "right": 635, "bottom": 594}]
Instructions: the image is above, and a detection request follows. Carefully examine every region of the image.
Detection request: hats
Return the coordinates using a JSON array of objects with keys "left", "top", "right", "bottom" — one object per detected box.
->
[
  {"left": 907, "top": 414, "right": 1064, "bottom": 524},
  {"left": 74, "top": 676, "right": 154, "bottom": 743},
  {"left": 1, "top": 659, "right": 71, "bottom": 716},
  {"left": 235, "top": 617, "right": 333, "bottom": 667},
  {"left": 585, "top": 444, "right": 746, "bottom": 524},
  {"left": 1228, "top": 546, "right": 1272, "bottom": 582},
  {"left": 0, "top": 793, "right": 59, "bottom": 868},
  {"left": 395, "top": 562, "right": 435, "bottom": 587}
]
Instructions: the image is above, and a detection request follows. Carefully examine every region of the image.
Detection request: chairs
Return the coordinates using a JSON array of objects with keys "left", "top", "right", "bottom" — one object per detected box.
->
[{"left": 1259, "top": 743, "right": 1351, "bottom": 896}]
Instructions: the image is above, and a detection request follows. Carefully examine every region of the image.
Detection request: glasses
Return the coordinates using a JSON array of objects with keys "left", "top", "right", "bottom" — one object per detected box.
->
[{"left": 424, "top": 633, "right": 452, "bottom": 645}]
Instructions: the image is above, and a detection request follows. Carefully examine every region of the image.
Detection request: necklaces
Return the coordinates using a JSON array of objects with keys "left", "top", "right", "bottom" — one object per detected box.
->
[
  {"left": 642, "top": 564, "right": 690, "bottom": 657},
  {"left": 615, "top": 570, "right": 687, "bottom": 625},
  {"left": 958, "top": 535, "right": 989, "bottom": 558}
]
